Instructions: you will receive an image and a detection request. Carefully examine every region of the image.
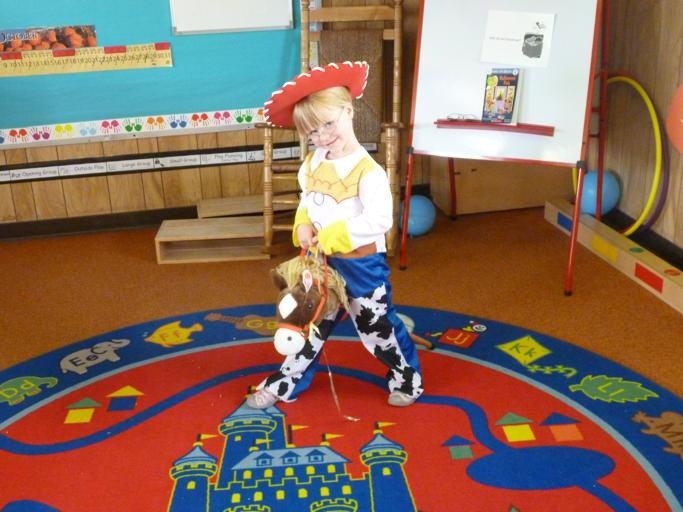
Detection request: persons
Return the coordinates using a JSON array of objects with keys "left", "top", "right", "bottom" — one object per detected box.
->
[
  {"left": 244, "top": 60, "right": 424, "bottom": 409},
  {"left": 0, "top": 25, "right": 96, "bottom": 52}
]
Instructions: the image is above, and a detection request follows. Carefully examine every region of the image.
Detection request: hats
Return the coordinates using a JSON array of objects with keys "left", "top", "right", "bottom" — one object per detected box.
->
[{"left": 264, "top": 60, "right": 370, "bottom": 130}]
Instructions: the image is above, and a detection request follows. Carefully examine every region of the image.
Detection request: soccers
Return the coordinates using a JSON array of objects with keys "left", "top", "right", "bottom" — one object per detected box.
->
[
  {"left": 399, "top": 196, "right": 436, "bottom": 236},
  {"left": 580, "top": 169, "right": 620, "bottom": 216}
]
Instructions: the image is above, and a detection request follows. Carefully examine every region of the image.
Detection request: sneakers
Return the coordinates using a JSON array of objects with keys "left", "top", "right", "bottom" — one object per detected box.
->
[
  {"left": 388, "top": 384, "right": 420, "bottom": 407},
  {"left": 246, "top": 381, "right": 284, "bottom": 411}
]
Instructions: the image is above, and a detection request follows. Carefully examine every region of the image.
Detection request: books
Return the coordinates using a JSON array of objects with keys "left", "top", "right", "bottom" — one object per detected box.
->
[{"left": 480, "top": 67, "right": 524, "bottom": 127}]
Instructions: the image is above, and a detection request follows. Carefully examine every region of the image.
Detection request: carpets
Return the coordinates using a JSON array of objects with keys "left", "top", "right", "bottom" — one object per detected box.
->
[{"left": 0, "top": 299, "right": 683, "bottom": 512}]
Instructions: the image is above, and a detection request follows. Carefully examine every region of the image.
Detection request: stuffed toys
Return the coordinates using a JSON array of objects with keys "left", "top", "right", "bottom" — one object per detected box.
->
[{"left": 267, "top": 246, "right": 351, "bottom": 357}]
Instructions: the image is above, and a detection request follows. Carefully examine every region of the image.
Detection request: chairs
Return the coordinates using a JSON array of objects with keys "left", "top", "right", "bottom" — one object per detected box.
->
[{"left": 256, "top": 0, "right": 406, "bottom": 263}]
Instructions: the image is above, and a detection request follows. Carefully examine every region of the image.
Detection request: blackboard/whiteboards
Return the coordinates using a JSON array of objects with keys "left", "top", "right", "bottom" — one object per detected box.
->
[{"left": 405, "top": 0, "right": 603, "bottom": 169}]
